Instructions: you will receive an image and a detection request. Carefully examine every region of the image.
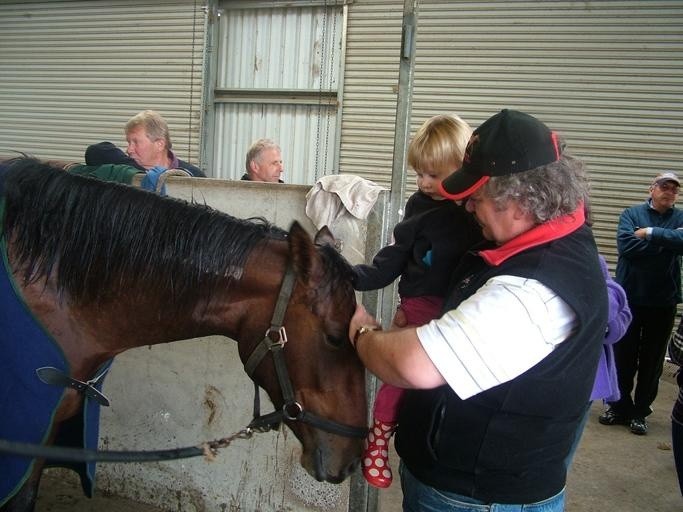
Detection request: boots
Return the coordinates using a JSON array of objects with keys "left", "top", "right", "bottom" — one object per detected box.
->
[{"left": 361, "top": 417, "right": 399, "bottom": 489}]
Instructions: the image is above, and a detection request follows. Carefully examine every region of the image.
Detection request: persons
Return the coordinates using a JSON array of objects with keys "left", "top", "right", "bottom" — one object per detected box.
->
[
  {"left": 668, "top": 313, "right": 683, "bottom": 496},
  {"left": 241, "top": 139, "right": 284, "bottom": 183},
  {"left": 351, "top": 113, "right": 490, "bottom": 488},
  {"left": 349, "top": 109, "right": 609, "bottom": 512},
  {"left": 563, "top": 255, "right": 633, "bottom": 463},
  {"left": 85, "top": 110, "right": 205, "bottom": 177},
  {"left": 598, "top": 173, "right": 682, "bottom": 436}
]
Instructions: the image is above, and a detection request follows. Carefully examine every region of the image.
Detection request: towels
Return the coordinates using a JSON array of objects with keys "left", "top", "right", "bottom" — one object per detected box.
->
[{"left": 305, "top": 175, "right": 389, "bottom": 230}]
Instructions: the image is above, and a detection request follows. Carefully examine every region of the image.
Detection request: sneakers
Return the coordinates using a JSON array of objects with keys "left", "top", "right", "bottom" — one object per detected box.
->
[
  {"left": 598, "top": 407, "right": 632, "bottom": 425},
  {"left": 630, "top": 416, "right": 648, "bottom": 434}
]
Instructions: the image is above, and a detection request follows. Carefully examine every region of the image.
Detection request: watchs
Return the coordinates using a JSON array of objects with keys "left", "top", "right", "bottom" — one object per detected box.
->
[{"left": 354, "top": 324, "right": 382, "bottom": 351}]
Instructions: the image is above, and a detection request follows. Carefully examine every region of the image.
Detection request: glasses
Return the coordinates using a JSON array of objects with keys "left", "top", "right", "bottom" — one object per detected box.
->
[{"left": 659, "top": 183, "right": 680, "bottom": 194}]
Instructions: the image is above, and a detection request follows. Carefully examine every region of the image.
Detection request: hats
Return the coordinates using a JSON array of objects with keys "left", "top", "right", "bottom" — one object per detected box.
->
[
  {"left": 651, "top": 173, "right": 682, "bottom": 188},
  {"left": 439, "top": 109, "right": 566, "bottom": 202}
]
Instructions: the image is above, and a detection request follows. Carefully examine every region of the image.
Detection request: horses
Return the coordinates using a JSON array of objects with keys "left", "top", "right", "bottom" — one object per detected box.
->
[{"left": 0, "top": 149, "right": 369, "bottom": 512}]
